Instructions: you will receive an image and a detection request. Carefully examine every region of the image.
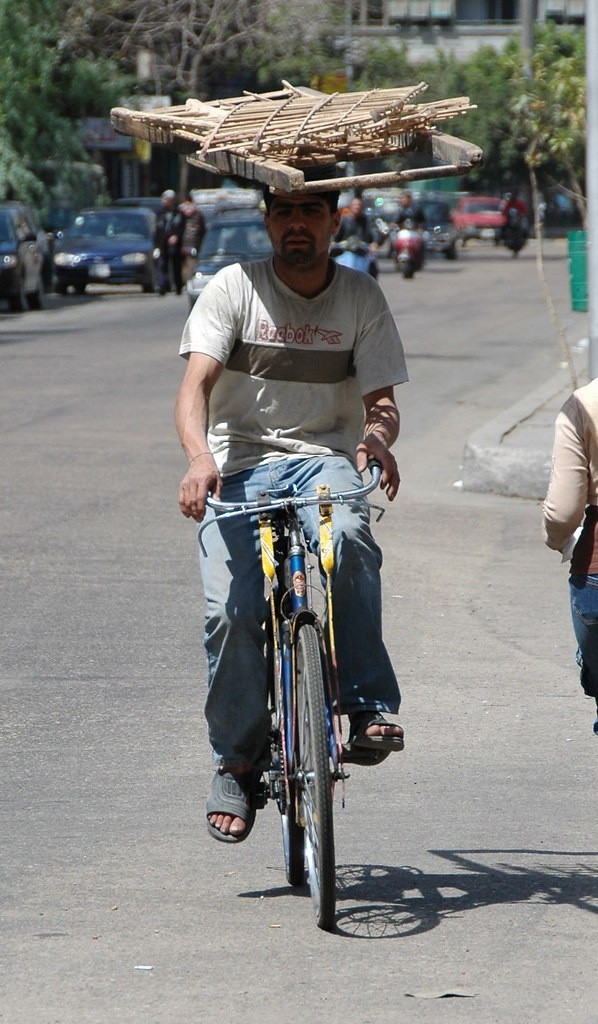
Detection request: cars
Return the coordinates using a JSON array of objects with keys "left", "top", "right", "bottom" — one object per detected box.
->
[
  {"left": 454, "top": 195, "right": 507, "bottom": 247},
  {"left": 186, "top": 208, "right": 278, "bottom": 302},
  {"left": 0, "top": 200, "right": 52, "bottom": 314},
  {"left": 51, "top": 204, "right": 165, "bottom": 298},
  {"left": 420, "top": 202, "right": 467, "bottom": 261}
]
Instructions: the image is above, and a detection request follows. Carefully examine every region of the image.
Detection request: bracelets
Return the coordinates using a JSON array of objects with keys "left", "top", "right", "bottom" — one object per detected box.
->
[{"left": 187, "top": 451, "right": 214, "bottom": 466}]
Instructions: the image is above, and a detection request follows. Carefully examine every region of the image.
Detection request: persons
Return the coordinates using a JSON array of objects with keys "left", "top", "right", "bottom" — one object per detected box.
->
[
  {"left": 389, "top": 190, "right": 427, "bottom": 242},
  {"left": 173, "top": 169, "right": 410, "bottom": 844},
  {"left": 330, "top": 199, "right": 382, "bottom": 283},
  {"left": 153, "top": 188, "right": 204, "bottom": 296},
  {"left": 497, "top": 192, "right": 530, "bottom": 244},
  {"left": 543, "top": 377, "right": 598, "bottom": 735}
]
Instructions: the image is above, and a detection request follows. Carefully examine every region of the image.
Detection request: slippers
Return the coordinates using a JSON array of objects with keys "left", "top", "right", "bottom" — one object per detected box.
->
[
  {"left": 205, "top": 767, "right": 263, "bottom": 843},
  {"left": 347, "top": 713, "right": 405, "bottom": 751}
]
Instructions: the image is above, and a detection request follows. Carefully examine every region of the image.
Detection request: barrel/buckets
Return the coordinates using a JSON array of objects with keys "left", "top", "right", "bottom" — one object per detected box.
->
[{"left": 568, "top": 232, "right": 587, "bottom": 312}]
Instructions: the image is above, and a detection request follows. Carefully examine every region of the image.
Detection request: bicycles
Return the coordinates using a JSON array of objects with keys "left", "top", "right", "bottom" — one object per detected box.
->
[{"left": 197, "top": 459, "right": 384, "bottom": 929}]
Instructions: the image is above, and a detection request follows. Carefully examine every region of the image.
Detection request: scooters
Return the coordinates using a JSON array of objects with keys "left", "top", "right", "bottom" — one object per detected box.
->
[
  {"left": 503, "top": 208, "right": 529, "bottom": 255},
  {"left": 391, "top": 219, "right": 430, "bottom": 275},
  {"left": 337, "top": 237, "right": 381, "bottom": 283}
]
